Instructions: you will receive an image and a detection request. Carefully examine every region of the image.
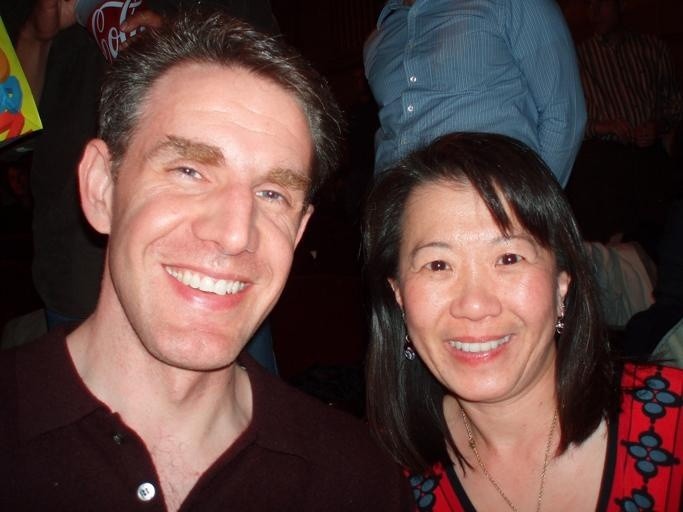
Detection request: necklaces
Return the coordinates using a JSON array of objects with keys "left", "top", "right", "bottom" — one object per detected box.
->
[{"left": 457, "top": 405, "right": 564, "bottom": 511}]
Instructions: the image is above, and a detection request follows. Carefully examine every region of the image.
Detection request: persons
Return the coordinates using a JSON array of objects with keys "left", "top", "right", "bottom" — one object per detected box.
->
[
  {"left": 0, "top": 18, "right": 419, "bottom": 512},
  {"left": 561, "top": 0, "right": 681, "bottom": 323},
  {"left": 360, "top": 127, "right": 681, "bottom": 512},
  {"left": 0, "top": 0, "right": 286, "bottom": 374},
  {"left": 358, "top": 1, "right": 588, "bottom": 192}
]
